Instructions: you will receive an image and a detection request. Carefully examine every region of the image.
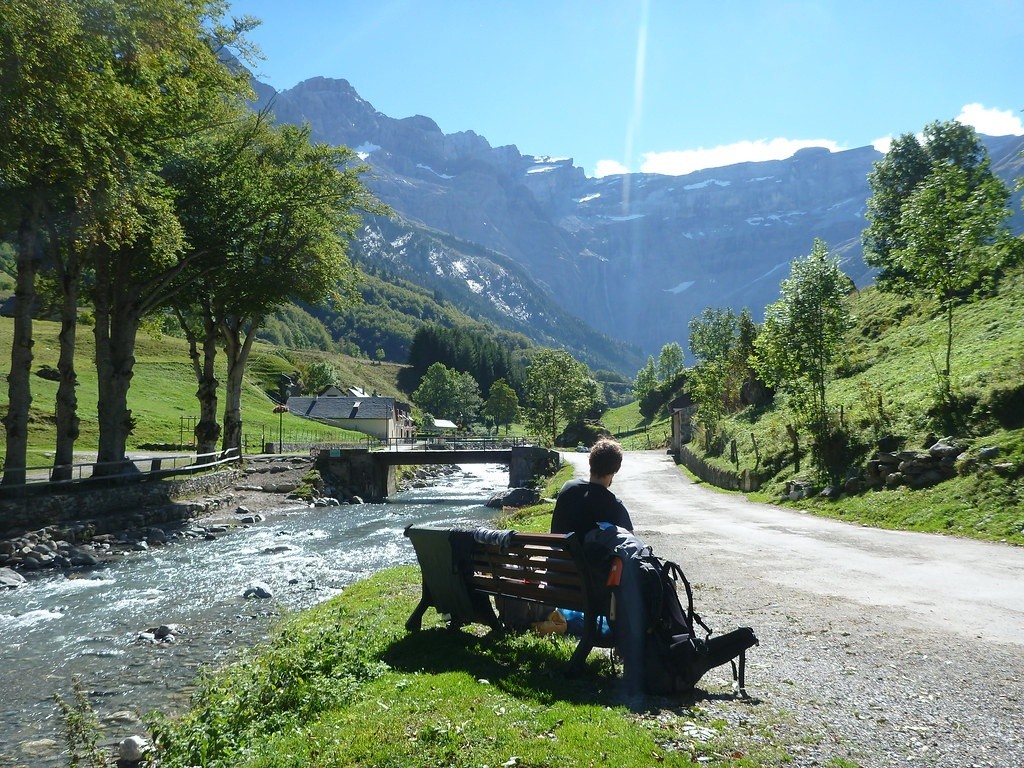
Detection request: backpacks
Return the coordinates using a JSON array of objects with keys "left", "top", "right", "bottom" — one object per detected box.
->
[{"left": 585, "top": 526, "right": 706, "bottom": 694}]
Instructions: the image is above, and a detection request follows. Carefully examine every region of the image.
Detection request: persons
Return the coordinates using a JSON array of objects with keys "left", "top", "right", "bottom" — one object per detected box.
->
[{"left": 546, "top": 439, "right": 634, "bottom": 588}]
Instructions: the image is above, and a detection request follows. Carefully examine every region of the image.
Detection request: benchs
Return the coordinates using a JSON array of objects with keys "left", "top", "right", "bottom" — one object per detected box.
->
[{"left": 404, "top": 523, "right": 756, "bottom": 693}]
